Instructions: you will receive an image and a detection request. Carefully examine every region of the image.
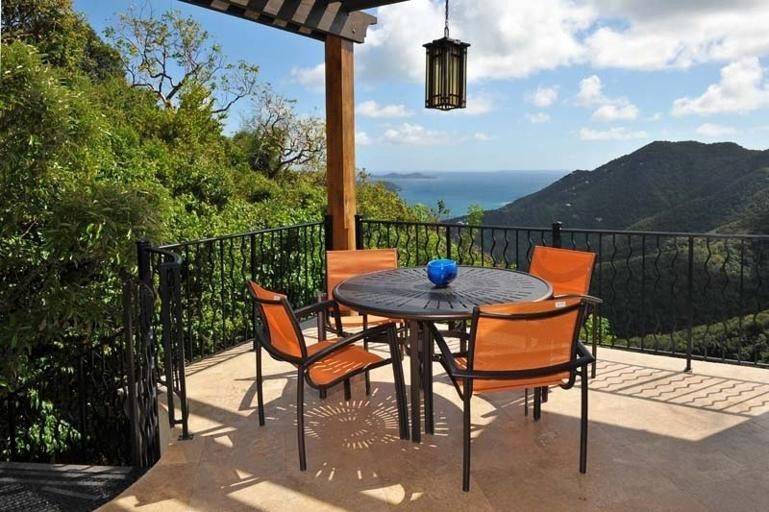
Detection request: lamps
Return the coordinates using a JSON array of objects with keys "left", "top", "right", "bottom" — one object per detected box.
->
[{"left": 423, "top": 0, "right": 470, "bottom": 111}]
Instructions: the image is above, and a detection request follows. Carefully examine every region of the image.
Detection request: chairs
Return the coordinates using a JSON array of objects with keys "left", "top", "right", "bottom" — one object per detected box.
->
[
  {"left": 314, "top": 247, "right": 399, "bottom": 402},
  {"left": 422, "top": 293, "right": 603, "bottom": 492},
  {"left": 245, "top": 279, "right": 410, "bottom": 472},
  {"left": 518, "top": 244, "right": 603, "bottom": 419}
]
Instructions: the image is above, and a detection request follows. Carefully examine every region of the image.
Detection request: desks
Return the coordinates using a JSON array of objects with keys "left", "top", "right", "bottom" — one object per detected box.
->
[{"left": 329, "top": 264, "right": 554, "bottom": 444}]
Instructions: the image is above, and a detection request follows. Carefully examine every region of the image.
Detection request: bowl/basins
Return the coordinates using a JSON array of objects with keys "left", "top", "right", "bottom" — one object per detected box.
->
[{"left": 426, "top": 259, "right": 458, "bottom": 289}]
